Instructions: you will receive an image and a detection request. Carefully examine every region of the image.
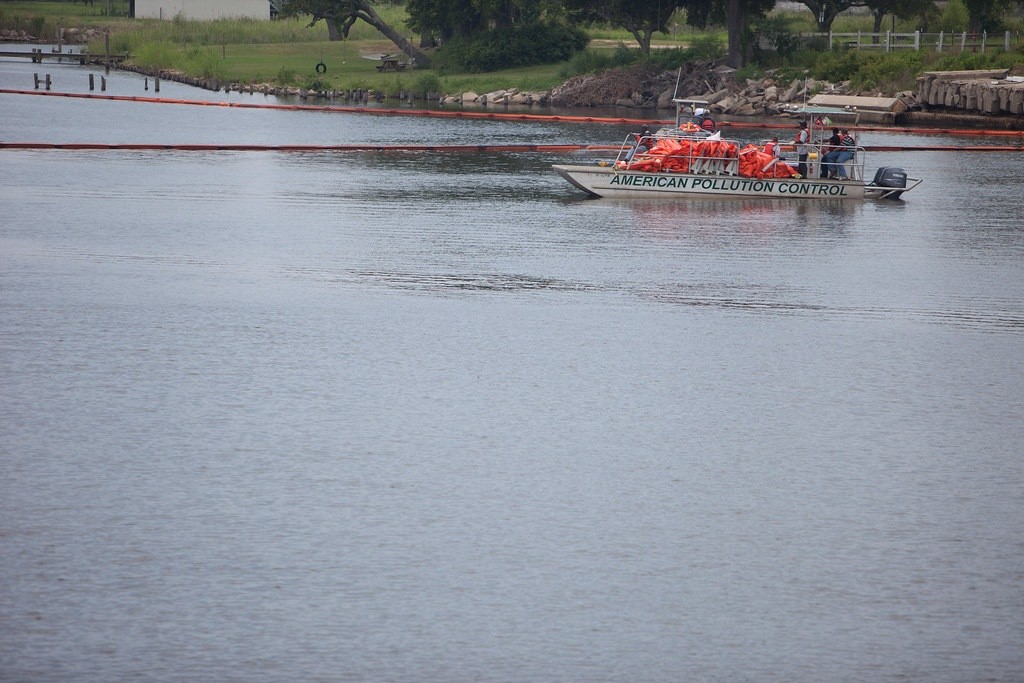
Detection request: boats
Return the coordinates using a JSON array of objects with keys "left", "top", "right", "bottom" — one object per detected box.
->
[{"left": 552, "top": 98, "right": 923, "bottom": 200}]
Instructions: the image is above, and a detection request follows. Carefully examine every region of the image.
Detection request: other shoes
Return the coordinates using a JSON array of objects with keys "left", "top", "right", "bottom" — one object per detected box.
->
[
  {"left": 836, "top": 176, "right": 847, "bottom": 179},
  {"left": 829, "top": 168, "right": 836, "bottom": 179},
  {"left": 820, "top": 172, "right": 828, "bottom": 177}
]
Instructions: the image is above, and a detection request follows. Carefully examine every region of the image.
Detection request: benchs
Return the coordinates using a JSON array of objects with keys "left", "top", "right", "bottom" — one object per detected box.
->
[
  {"left": 376, "top": 65, "right": 383, "bottom": 69},
  {"left": 399, "top": 64, "right": 405, "bottom": 68},
  {"left": 833, "top": 159, "right": 854, "bottom": 179}
]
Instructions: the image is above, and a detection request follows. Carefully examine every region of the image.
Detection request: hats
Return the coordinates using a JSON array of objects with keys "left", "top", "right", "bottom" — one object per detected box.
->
[
  {"left": 798, "top": 121, "right": 807, "bottom": 127},
  {"left": 842, "top": 128, "right": 848, "bottom": 134}
]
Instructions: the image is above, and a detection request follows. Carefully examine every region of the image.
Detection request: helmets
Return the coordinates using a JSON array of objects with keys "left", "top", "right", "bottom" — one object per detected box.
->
[{"left": 694, "top": 108, "right": 706, "bottom": 115}]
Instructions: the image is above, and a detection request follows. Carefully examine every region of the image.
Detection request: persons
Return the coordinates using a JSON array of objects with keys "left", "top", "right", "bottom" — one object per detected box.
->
[
  {"left": 764, "top": 137, "right": 781, "bottom": 157},
  {"left": 818, "top": 127, "right": 856, "bottom": 180},
  {"left": 622, "top": 124, "right": 653, "bottom": 165},
  {"left": 700, "top": 111, "right": 715, "bottom": 131},
  {"left": 793, "top": 121, "right": 810, "bottom": 179}
]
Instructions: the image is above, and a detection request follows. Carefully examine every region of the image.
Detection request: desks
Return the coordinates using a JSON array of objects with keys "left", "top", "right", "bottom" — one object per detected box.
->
[{"left": 380, "top": 58, "right": 401, "bottom": 72}]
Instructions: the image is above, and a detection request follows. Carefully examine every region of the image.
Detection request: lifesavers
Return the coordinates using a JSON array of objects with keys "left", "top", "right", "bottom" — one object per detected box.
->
[
  {"left": 316, "top": 63, "right": 326, "bottom": 74},
  {"left": 679, "top": 123, "right": 702, "bottom": 132}
]
